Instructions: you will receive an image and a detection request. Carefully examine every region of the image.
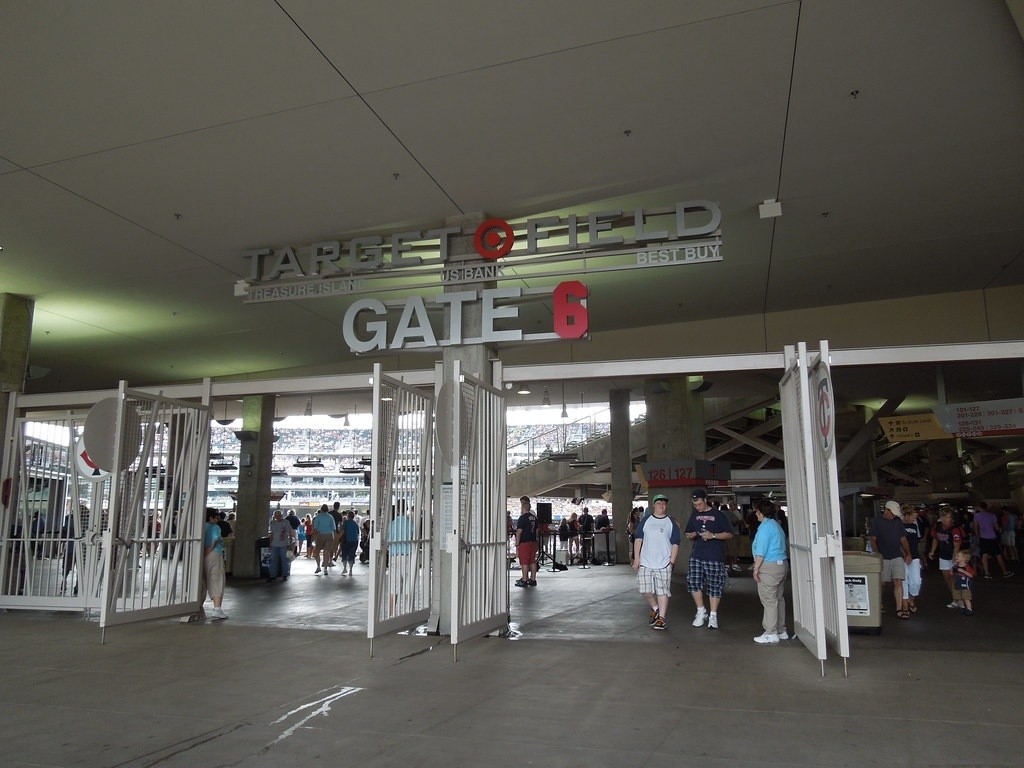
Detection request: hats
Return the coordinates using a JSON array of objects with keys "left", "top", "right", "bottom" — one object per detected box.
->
[
  {"left": 884, "top": 500, "right": 901, "bottom": 516},
  {"left": 690, "top": 489, "right": 709, "bottom": 499},
  {"left": 652, "top": 493, "right": 669, "bottom": 503}
]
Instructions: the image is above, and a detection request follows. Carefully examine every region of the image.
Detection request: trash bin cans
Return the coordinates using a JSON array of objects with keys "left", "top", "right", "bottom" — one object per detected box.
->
[
  {"left": 258, "top": 537, "right": 271, "bottom": 579},
  {"left": 844, "top": 553, "right": 884, "bottom": 636},
  {"left": 222, "top": 537, "right": 234, "bottom": 577}
]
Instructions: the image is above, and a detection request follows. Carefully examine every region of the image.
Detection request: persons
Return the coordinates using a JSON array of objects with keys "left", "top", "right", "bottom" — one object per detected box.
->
[
  {"left": 559, "top": 508, "right": 609, "bottom": 553},
  {"left": 628, "top": 507, "right": 644, "bottom": 566},
  {"left": 338, "top": 511, "right": 359, "bottom": 576},
  {"left": 298, "top": 506, "right": 370, "bottom": 563},
  {"left": 684, "top": 489, "right": 733, "bottom": 630},
  {"left": 285, "top": 509, "right": 300, "bottom": 555},
  {"left": 33, "top": 511, "right": 45, "bottom": 559},
  {"left": 311, "top": 504, "right": 336, "bottom": 574},
  {"left": 139, "top": 511, "right": 178, "bottom": 558},
  {"left": 60, "top": 505, "right": 89, "bottom": 594},
  {"left": 515, "top": 496, "right": 538, "bottom": 587},
  {"left": 322, "top": 502, "right": 343, "bottom": 566},
  {"left": 632, "top": 494, "right": 680, "bottom": 630},
  {"left": 864, "top": 501, "right": 1020, "bottom": 620},
  {"left": 267, "top": 510, "right": 292, "bottom": 583},
  {"left": 751, "top": 499, "right": 788, "bottom": 643},
  {"left": 508, "top": 511, "right": 513, "bottom": 530},
  {"left": 384, "top": 499, "right": 423, "bottom": 615},
  {"left": 203, "top": 508, "right": 235, "bottom": 619},
  {"left": 711, "top": 500, "right": 788, "bottom": 572}
]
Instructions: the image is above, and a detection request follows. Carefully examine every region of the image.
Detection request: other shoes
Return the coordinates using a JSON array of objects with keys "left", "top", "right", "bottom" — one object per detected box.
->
[
  {"left": 947, "top": 602, "right": 974, "bottom": 616},
  {"left": 61, "top": 582, "right": 67, "bottom": 591},
  {"left": 1010, "top": 557, "right": 1019, "bottom": 561},
  {"left": 984, "top": 574, "right": 993, "bottom": 579},
  {"left": 284, "top": 576, "right": 288, "bottom": 581},
  {"left": 266, "top": 576, "right": 276, "bottom": 583},
  {"left": 896, "top": 604, "right": 918, "bottom": 619},
  {"left": 314, "top": 568, "right": 322, "bottom": 573},
  {"left": 881, "top": 601, "right": 886, "bottom": 614},
  {"left": 1003, "top": 571, "right": 1015, "bottom": 578},
  {"left": 74, "top": 586, "right": 78, "bottom": 594},
  {"left": 323, "top": 570, "right": 328, "bottom": 575},
  {"left": 515, "top": 579, "right": 538, "bottom": 587},
  {"left": 340, "top": 569, "right": 353, "bottom": 577},
  {"left": 322, "top": 562, "right": 336, "bottom": 567}
]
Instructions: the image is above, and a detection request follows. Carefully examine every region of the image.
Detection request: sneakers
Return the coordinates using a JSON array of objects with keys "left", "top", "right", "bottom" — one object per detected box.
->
[
  {"left": 211, "top": 609, "right": 229, "bottom": 620},
  {"left": 778, "top": 632, "right": 789, "bottom": 640},
  {"left": 648, "top": 607, "right": 660, "bottom": 626},
  {"left": 707, "top": 615, "right": 719, "bottom": 628},
  {"left": 692, "top": 607, "right": 709, "bottom": 628},
  {"left": 199, "top": 606, "right": 209, "bottom": 619},
  {"left": 653, "top": 616, "right": 668, "bottom": 630},
  {"left": 753, "top": 633, "right": 780, "bottom": 644}
]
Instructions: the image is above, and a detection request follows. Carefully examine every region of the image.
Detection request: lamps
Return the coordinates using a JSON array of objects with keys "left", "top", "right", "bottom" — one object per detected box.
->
[
  {"left": 518, "top": 384, "right": 530, "bottom": 394},
  {"left": 541, "top": 380, "right": 551, "bottom": 407},
  {"left": 344, "top": 414, "right": 349, "bottom": 426},
  {"left": 561, "top": 382, "right": 568, "bottom": 418},
  {"left": 304, "top": 395, "right": 312, "bottom": 416}
]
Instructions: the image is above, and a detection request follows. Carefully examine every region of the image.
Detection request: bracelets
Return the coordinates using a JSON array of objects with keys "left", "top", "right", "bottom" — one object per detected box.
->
[
  {"left": 515, "top": 545, "right": 519, "bottom": 547},
  {"left": 713, "top": 533, "right": 715, "bottom": 539}
]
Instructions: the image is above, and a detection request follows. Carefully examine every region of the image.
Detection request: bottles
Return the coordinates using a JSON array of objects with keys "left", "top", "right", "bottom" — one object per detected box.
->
[
  {"left": 700, "top": 519, "right": 707, "bottom": 541},
  {"left": 866, "top": 530, "right": 870, "bottom": 541}
]
